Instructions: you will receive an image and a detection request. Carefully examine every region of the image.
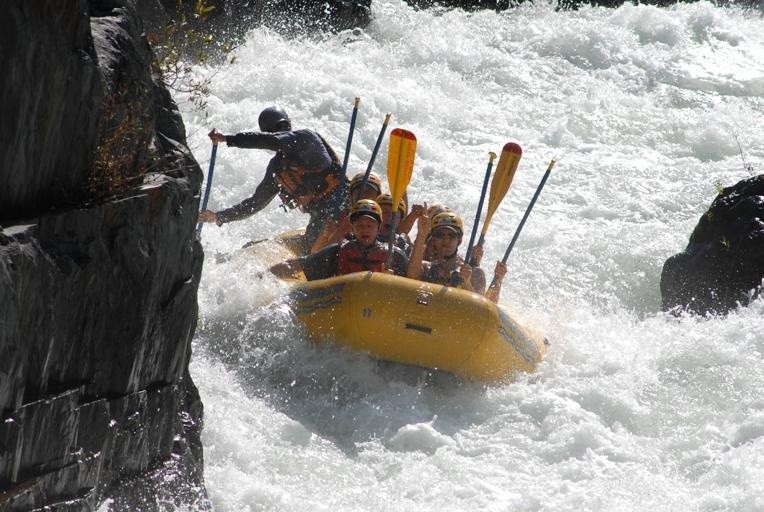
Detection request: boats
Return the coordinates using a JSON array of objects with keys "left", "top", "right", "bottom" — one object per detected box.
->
[{"left": 233, "top": 228, "right": 551, "bottom": 384}]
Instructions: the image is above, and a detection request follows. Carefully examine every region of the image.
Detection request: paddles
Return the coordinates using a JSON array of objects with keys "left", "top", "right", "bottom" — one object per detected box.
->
[
  {"left": 469, "top": 143, "right": 522, "bottom": 264},
  {"left": 385, "top": 130, "right": 416, "bottom": 271},
  {"left": 311, "top": 97, "right": 389, "bottom": 251}
]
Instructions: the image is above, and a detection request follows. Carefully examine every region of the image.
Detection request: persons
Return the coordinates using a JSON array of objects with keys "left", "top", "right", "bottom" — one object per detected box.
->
[
  {"left": 268, "top": 170, "right": 507, "bottom": 304},
  {"left": 196, "top": 105, "right": 352, "bottom": 255}
]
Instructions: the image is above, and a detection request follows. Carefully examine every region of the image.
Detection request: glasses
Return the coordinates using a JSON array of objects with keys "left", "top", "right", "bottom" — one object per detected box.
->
[{"left": 434, "top": 231, "right": 461, "bottom": 237}]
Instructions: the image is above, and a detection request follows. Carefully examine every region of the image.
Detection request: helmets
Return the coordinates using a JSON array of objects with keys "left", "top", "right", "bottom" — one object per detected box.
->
[
  {"left": 425, "top": 205, "right": 452, "bottom": 243},
  {"left": 259, "top": 107, "right": 290, "bottom": 132},
  {"left": 350, "top": 173, "right": 382, "bottom": 194},
  {"left": 349, "top": 200, "right": 383, "bottom": 224},
  {"left": 431, "top": 213, "right": 464, "bottom": 239},
  {"left": 376, "top": 193, "right": 406, "bottom": 221}
]
